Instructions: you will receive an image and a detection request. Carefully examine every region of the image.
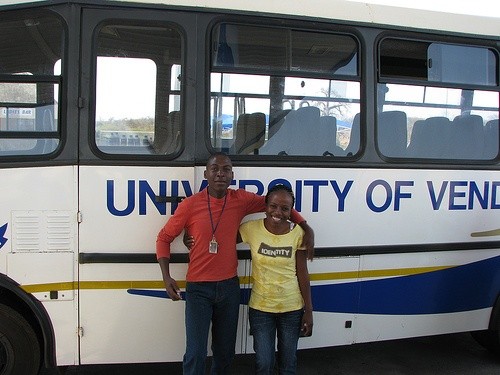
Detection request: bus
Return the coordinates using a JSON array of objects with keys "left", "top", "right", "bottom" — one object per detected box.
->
[{"left": 0, "top": 0, "right": 500, "bottom": 375}]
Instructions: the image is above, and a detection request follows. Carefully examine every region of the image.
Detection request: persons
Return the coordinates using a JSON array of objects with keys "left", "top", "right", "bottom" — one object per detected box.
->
[
  {"left": 108, "top": 133, "right": 154, "bottom": 146},
  {"left": 156, "top": 153, "right": 314, "bottom": 375},
  {"left": 183, "top": 184, "right": 313, "bottom": 375}
]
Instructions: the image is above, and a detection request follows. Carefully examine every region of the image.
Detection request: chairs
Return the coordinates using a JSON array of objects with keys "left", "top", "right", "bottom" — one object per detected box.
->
[{"left": 40, "top": 108, "right": 500, "bottom": 164}]
